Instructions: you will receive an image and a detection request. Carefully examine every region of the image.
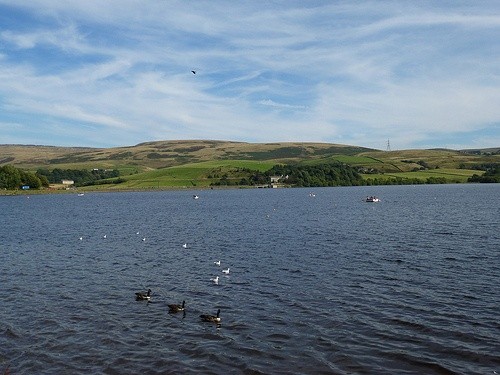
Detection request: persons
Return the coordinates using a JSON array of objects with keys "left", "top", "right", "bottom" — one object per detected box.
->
[{"left": 367, "top": 196, "right": 376, "bottom": 200}]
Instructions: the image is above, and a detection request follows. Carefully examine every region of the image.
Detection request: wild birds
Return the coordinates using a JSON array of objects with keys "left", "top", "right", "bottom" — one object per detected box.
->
[
  {"left": 78, "top": 236, "right": 83, "bottom": 240},
  {"left": 210, "top": 276, "right": 220, "bottom": 284},
  {"left": 102, "top": 234, "right": 107, "bottom": 239},
  {"left": 182, "top": 243, "right": 187, "bottom": 248},
  {"left": 308, "top": 191, "right": 317, "bottom": 197},
  {"left": 222, "top": 267, "right": 230, "bottom": 274},
  {"left": 136, "top": 231, "right": 140, "bottom": 235},
  {"left": 141, "top": 237, "right": 146, "bottom": 241},
  {"left": 192, "top": 194, "right": 200, "bottom": 200},
  {"left": 190, "top": 69, "right": 196, "bottom": 75},
  {"left": 285, "top": 174, "right": 289, "bottom": 179},
  {"left": 213, "top": 260, "right": 221, "bottom": 267}
]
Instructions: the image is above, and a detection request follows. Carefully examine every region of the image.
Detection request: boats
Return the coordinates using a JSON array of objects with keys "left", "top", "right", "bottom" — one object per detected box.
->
[
  {"left": 78, "top": 193, "right": 85, "bottom": 197},
  {"left": 365, "top": 197, "right": 381, "bottom": 202}
]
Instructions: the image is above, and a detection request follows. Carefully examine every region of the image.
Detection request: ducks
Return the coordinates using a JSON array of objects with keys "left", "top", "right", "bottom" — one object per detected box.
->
[
  {"left": 167, "top": 300, "right": 187, "bottom": 311},
  {"left": 134, "top": 288, "right": 153, "bottom": 299},
  {"left": 199, "top": 308, "right": 222, "bottom": 323}
]
